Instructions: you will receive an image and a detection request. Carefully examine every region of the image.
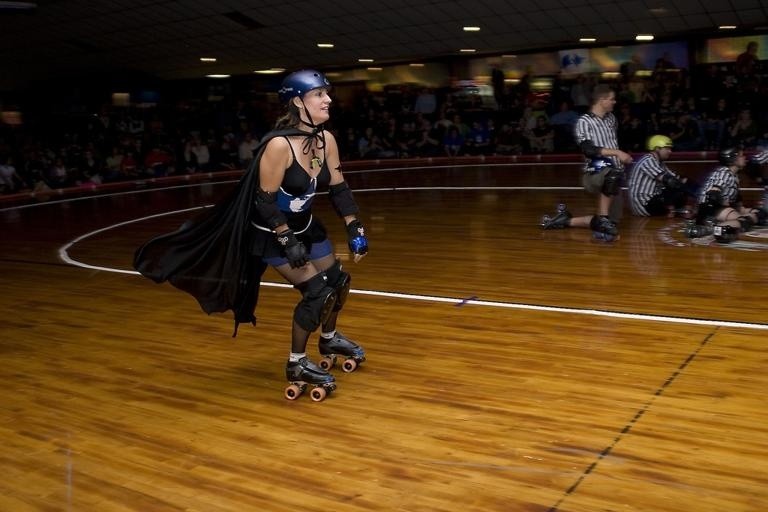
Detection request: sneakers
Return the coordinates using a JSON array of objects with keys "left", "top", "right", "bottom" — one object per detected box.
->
[{"left": 687, "top": 225, "right": 713, "bottom": 238}]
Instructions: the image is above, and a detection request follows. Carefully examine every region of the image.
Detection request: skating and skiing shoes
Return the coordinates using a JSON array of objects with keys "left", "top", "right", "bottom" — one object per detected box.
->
[
  {"left": 590, "top": 215, "right": 622, "bottom": 242},
  {"left": 317, "top": 330, "right": 365, "bottom": 374},
  {"left": 284, "top": 356, "right": 336, "bottom": 402},
  {"left": 541, "top": 203, "right": 572, "bottom": 229},
  {"left": 665, "top": 203, "right": 696, "bottom": 220}
]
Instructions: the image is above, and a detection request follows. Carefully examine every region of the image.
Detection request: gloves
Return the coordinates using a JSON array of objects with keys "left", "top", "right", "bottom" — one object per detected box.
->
[
  {"left": 277, "top": 228, "right": 310, "bottom": 270},
  {"left": 346, "top": 218, "right": 370, "bottom": 255}
]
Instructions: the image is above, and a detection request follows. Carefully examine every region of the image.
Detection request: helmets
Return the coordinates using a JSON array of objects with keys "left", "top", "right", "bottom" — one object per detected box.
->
[
  {"left": 648, "top": 135, "right": 672, "bottom": 151},
  {"left": 279, "top": 70, "right": 328, "bottom": 106},
  {"left": 718, "top": 142, "right": 745, "bottom": 165}
]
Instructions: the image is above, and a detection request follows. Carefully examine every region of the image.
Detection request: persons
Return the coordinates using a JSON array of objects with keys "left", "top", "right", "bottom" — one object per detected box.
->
[
  {"left": 692, "top": 145, "right": 768, "bottom": 243},
  {"left": 541, "top": 82, "right": 634, "bottom": 236},
  {"left": 241, "top": 68, "right": 370, "bottom": 387},
  {"left": 627, "top": 132, "right": 698, "bottom": 221},
  {"left": 739, "top": 148, "right": 768, "bottom": 215},
  {"left": 1, "top": 38, "right": 768, "bottom": 198}
]
